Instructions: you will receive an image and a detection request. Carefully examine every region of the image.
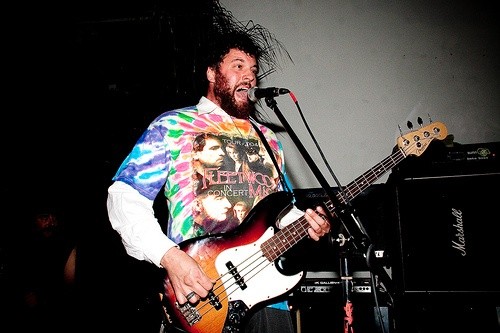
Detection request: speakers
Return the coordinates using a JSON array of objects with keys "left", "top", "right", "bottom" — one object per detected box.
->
[
  {"left": 393, "top": 142, "right": 500, "bottom": 297},
  {"left": 392, "top": 289, "right": 500, "bottom": 333},
  {"left": 288, "top": 276, "right": 393, "bottom": 333},
  {"left": 290, "top": 184, "right": 403, "bottom": 279}
]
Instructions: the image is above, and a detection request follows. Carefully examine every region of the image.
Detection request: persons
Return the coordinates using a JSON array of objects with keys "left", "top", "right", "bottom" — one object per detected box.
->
[
  {"left": 0, "top": 204, "right": 77, "bottom": 333},
  {"left": 106, "top": 32, "right": 331, "bottom": 333}
]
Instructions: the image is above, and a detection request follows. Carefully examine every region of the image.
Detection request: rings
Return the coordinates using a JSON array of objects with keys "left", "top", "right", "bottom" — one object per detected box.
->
[{"left": 186, "top": 291, "right": 196, "bottom": 301}]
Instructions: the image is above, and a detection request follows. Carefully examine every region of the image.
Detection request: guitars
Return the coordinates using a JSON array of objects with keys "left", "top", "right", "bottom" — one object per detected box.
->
[{"left": 158, "top": 112, "right": 450, "bottom": 333}]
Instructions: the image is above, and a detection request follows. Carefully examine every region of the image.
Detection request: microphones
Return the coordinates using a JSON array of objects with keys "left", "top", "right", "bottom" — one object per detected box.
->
[{"left": 248, "top": 86, "right": 288, "bottom": 102}]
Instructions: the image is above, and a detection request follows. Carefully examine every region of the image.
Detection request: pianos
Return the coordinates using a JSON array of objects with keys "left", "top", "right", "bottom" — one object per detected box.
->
[{"left": 292, "top": 269, "right": 372, "bottom": 299}]
